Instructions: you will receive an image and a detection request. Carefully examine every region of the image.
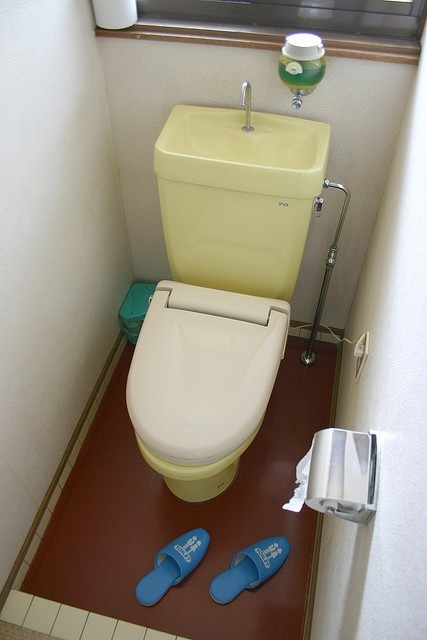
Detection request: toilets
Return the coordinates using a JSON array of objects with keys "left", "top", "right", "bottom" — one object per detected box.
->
[{"left": 124, "top": 102, "right": 334, "bottom": 505}]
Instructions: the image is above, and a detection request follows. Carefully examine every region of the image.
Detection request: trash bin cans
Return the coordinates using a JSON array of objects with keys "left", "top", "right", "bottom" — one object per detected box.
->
[{"left": 119, "top": 282, "right": 157, "bottom": 346}]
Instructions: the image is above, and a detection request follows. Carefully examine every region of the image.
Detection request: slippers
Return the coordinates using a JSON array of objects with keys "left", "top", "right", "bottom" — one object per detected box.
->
[
  {"left": 210, "top": 535, "right": 290, "bottom": 605},
  {"left": 136, "top": 529, "right": 209, "bottom": 607}
]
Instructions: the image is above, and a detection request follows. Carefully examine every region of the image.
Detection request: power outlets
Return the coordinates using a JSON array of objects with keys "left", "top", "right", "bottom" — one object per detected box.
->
[{"left": 355, "top": 331, "right": 369, "bottom": 383}]
